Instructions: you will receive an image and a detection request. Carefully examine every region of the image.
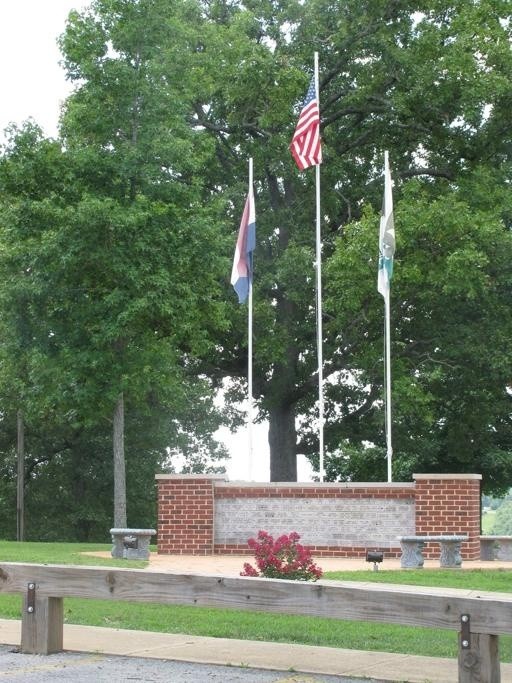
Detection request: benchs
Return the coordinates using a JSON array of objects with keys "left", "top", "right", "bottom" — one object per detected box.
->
[
  {"left": 475, "top": 534, "right": 512, "bottom": 566},
  {"left": 109, "top": 528, "right": 158, "bottom": 560},
  {"left": 399, "top": 534, "right": 470, "bottom": 569}
]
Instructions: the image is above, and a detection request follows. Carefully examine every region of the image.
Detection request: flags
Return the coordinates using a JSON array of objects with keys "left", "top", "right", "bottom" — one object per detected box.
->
[
  {"left": 377, "top": 160, "right": 396, "bottom": 297},
  {"left": 229, "top": 193, "right": 256, "bottom": 305},
  {"left": 290, "top": 74, "right": 323, "bottom": 171}
]
